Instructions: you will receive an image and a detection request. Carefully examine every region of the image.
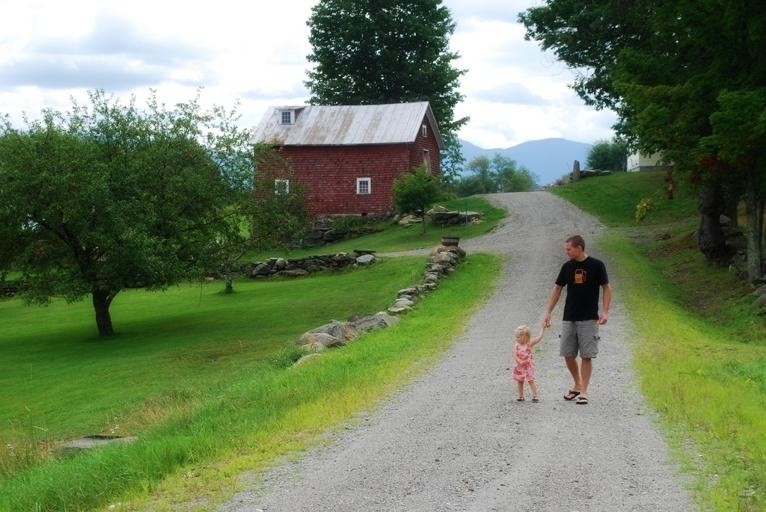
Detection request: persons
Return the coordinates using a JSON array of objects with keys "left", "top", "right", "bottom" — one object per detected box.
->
[
  {"left": 541, "top": 233, "right": 613, "bottom": 406},
  {"left": 510, "top": 321, "right": 550, "bottom": 402}
]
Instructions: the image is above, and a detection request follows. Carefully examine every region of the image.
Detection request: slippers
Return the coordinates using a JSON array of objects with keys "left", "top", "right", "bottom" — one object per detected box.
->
[
  {"left": 564, "top": 390, "right": 588, "bottom": 404},
  {"left": 517, "top": 395, "right": 539, "bottom": 401}
]
